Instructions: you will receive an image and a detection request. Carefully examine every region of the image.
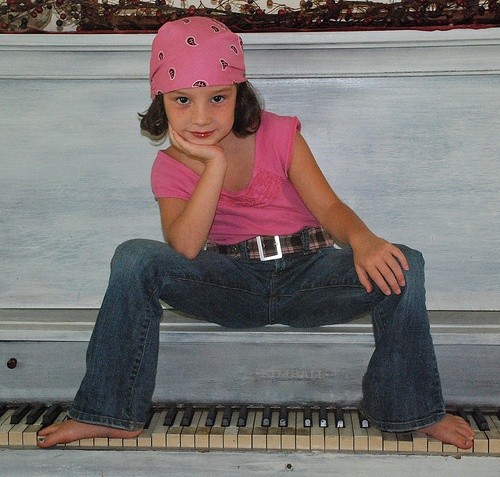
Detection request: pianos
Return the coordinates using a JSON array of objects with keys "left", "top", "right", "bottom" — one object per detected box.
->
[{"left": 0, "top": 27, "right": 500, "bottom": 475}]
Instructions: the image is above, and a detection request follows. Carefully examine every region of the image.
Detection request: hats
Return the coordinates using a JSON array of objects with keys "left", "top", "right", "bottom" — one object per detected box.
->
[{"left": 150, "top": 16, "right": 247, "bottom": 98}]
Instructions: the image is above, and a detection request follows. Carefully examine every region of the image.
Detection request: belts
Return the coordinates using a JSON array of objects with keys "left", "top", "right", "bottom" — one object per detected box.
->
[{"left": 206, "top": 227, "right": 338, "bottom": 263}]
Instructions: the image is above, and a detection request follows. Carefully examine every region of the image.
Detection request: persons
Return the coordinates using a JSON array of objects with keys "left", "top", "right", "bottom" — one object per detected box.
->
[{"left": 33, "top": 16, "right": 477, "bottom": 450}]
